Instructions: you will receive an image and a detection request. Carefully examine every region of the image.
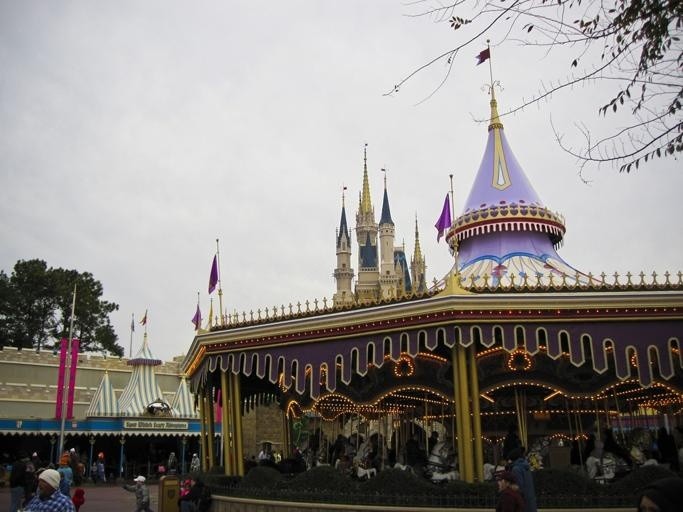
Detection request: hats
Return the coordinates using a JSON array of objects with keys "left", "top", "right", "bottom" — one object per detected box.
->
[
  {"left": 38, "top": 469, "right": 61, "bottom": 490},
  {"left": 134, "top": 475, "right": 146, "bottom": 482},
  {"left": 60, "top": 454, "right": 68, "bottom": 464}
]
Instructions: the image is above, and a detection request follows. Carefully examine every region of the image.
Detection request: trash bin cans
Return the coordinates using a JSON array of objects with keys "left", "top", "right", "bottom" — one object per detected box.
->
[{"left": 158, "top": 475, "right": 180, "bottom": 512}]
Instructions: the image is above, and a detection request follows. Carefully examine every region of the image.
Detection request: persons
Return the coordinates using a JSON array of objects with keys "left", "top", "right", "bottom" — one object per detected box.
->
[
  {"left": 189, "top": 453, "right": 201, "bottom": 474},
  {"left": 493, "top": 471, "right": 528, "bottom": 511},
  {"left": 155, "top": 451, "right": 187, "bottom": 476},
  {"left": 120, "top": 474, "right": 155, "bottom": 511},
  {"left": 242, "top": 441, "right": 306, "bottom": 476},
  {"left": 175, "top": 475, "right": 213, "bottom": 512},
  {"left": 0, "top": 445, "right": 89, "bottom": 512},
  {"left": 88, "top": 453, "right": 117, "bottom": 489},
  {"left": 493, "top": 425, "right": 682, "bottom": 468},
  {"left": 309, "top": 426, "right": 439, "bottom": 471},
  {"left": 496, "top": 445, "right": 537, "bottom": 512},
  {"left": 636, "top": 486, "right": 673, "bottom": 512}
]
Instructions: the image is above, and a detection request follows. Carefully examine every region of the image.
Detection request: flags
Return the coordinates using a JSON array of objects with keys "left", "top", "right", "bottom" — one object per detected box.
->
[
  {"left": 474, "top": 47, "right": 490, "bottom": 66},
  {"left": 191, "top": 304, "right": 202, "bottom": 331},
  {"left": 138, "top": 314, "right": 147, "bottom": 327},
  {"left": 208, "top": 254, "right": 219, "bottom": 295},
  {"left": 434, "top": 192, "right": 452, "bottom": 244}
]
